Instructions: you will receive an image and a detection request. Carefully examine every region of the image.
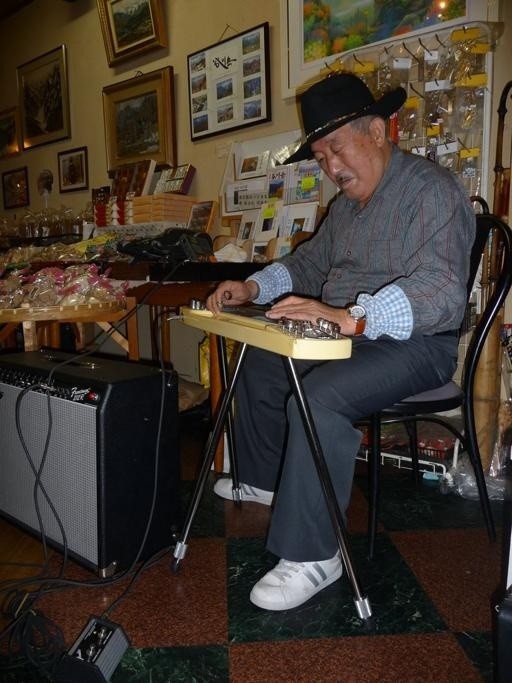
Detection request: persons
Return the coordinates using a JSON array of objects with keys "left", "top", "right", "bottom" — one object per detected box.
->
[
  {"left": 64, "top": 157, "right": 82, "bottom": 183},
  {"left": 204, "top": 68, "right": 478, "bottom": 613}
]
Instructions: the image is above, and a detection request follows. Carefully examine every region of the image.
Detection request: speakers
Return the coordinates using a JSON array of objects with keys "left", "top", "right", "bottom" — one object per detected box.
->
[{"left": 1, "top": 346, "right": 179, "bottom": 578}]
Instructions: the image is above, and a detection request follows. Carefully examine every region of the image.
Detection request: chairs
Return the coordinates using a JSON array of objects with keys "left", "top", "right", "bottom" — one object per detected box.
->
[
  {"left": 354, "top": 196, "right": 512, "bottom": 562},
  {"left": 127, "top": 281, "right": 227, "bottom": 473}
]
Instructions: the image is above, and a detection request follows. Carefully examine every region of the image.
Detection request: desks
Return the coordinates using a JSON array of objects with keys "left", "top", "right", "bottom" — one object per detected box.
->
[
  {"left": 0, "top": 261, "right": 272, "bottom": 361},
  {"left": 0, "top": 296, "right": 142, "bottom": 361}
]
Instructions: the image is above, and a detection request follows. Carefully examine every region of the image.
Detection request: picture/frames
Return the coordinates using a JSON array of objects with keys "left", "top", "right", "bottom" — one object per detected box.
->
[
  {"left": 0, "top": 105, "right": 24, "bottom": 161},
  {"left": 111, "top": 159, "right": 157, "bottom": 196},
  {"left": 187, "top": 21, "right": 272, "bottom": 142},
  {"left": 16, "top": 43, "right": 71, "bottom": 151},
  {"left": 57, "top": 146, "right": 89, "bottom": 193},
  {"left": 102, "top": 66, "right": 176, "bottom": 174},
  {"left": 96, "top": 0, "right": 169, "bottom": 67},
  {"left": 1, "top": 166, "right": 30, "bottom": 209},
  {"left": 279, "top": 0, "right": 500, "bottom": 102}
]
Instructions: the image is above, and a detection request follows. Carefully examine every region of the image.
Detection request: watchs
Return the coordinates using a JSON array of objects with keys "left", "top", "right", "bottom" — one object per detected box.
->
[{"left": 346, "top": 304, "right": 366, "bottom": 338}]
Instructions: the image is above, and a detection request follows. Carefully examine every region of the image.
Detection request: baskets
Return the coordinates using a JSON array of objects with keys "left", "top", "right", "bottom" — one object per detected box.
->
[{"left": 408, "top": 443, "right": 453, "bottom": 461}]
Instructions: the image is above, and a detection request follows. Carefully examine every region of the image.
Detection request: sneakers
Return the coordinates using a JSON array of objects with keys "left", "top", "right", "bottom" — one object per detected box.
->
[
  {"left": 249, "top": 550, "right": 344, "bottom": 610},
  {"left": 213, "top": 478, "right": 275, "bottom": 507}
]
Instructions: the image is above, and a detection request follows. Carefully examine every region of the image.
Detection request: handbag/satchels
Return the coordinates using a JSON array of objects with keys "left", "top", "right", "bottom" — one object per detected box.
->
[{"left": 117, "top": 227, "right": 213, "bottom": 259}]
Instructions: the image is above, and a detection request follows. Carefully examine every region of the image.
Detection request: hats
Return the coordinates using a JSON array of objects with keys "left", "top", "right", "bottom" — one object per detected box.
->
[{"left": 275, "top": 71, "right": 408, "bottom": 167}]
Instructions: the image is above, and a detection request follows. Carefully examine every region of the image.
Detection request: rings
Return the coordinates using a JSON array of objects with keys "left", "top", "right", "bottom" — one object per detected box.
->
[
  {"left": 224, "top": 291, "right": 232, "bottom": 300},
  {"left": 216, "top": 300, "right": 224, "bottom": 305}
]
